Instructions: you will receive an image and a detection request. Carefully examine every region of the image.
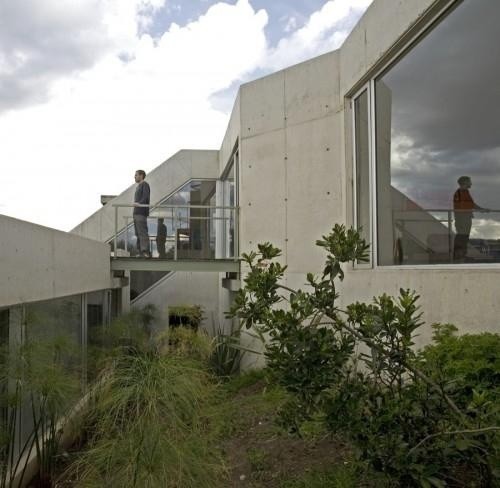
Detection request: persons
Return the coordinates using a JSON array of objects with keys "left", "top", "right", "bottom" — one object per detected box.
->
[
  {"left": 156, "top": 218, "right": 166, "bottom": 258},
  {"left": 453, "top": 177, "right": 489, "bottom": 260},
  {"left": 133, "top": 170, "right": 150, "bottom": 257}
]
